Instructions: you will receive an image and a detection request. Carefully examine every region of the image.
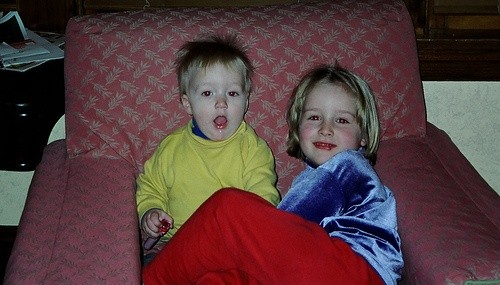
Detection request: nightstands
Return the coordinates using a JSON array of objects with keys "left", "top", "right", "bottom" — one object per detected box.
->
[{"left": 0, "top": 26, "right": 65, "bottom": 174}]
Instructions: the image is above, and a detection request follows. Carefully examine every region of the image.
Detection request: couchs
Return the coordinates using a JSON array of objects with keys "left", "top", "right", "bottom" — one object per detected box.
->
[{"left": 4, "top": 0, "right": 500, "bottom": 285}]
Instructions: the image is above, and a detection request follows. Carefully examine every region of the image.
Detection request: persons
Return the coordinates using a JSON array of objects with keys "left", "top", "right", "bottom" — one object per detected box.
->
[
  {"left": 135, "top": 34, "right": 281, "bottom": 265},
  {"left": 141, "top": 58, "right": 405, "bottom": 285}
]
